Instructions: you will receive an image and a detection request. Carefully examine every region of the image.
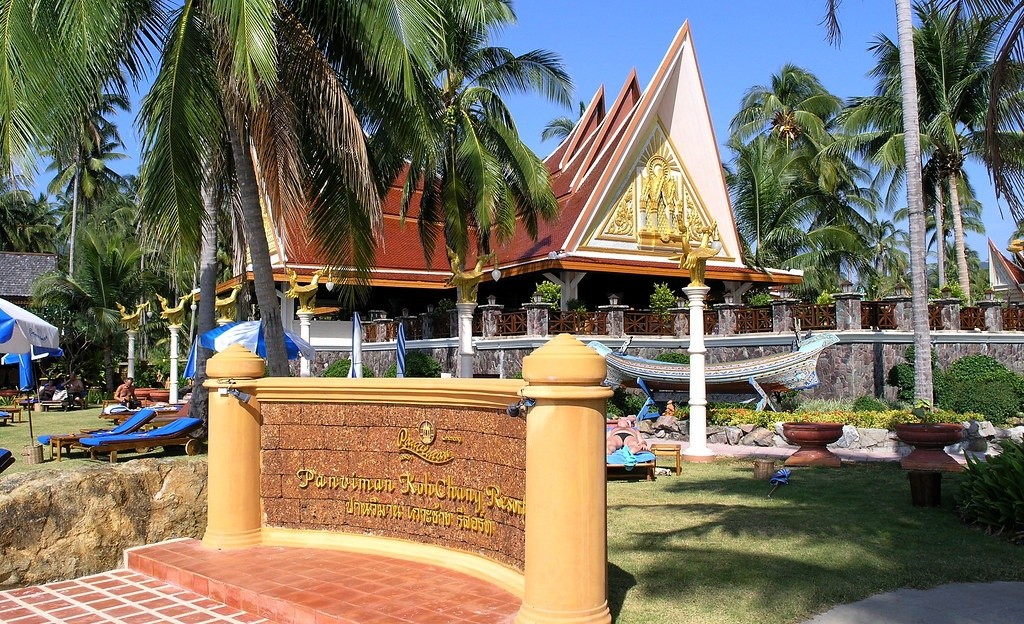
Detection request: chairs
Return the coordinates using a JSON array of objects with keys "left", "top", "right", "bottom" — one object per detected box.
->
[
  {"left": 607, "top": 420, "right": 656, "bottom": 483},
  {"left": 19, "top": 380, "right": 205, "bottom": 464}
]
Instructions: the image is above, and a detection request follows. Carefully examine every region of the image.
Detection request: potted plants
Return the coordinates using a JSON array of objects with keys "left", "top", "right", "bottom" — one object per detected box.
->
[
  {"left": 133, "top": 352, "right": 170, "bottom": 403},
  {"left": 569, "top": 299, "right": 595, "bottom": 335},
  {"left": 896, "top": 400, "right": 970, "bottom": 474}
]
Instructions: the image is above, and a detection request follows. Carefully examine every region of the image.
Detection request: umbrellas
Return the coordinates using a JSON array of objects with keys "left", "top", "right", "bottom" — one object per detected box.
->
[
  {"left": 396, "top": 323, "right": 406, "bottom": 378},
  {"left": 183, "top": 335, "right": 204, "bottom": 380},
  {"left": 197, "top": 318, "right": 316, "bottom": 360},
  {"left": 0, "top": 298, "right": 66, "bottom": 447},
  {"left": 347, "top": 311, "right": 362, "bottom": 378}
]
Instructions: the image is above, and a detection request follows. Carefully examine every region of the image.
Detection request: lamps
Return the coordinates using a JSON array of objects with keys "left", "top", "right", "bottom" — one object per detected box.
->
[
  {"left": 841, "top": 280, "right": 853, "bottom": 292},
  {"left": 779, "top": 286, "right": 790, "bottom": 298},
  {"left": 534, "top": 291, "right": 545, "bottom": 302},
  {"left": 402, "top": 308, "right": 409, "bottom": 317},
  {"left": 894, "top": 283, "right": 906, "bottom": 296},
  {"left": 427, "top": 304, "right": 434, "bottom": 313},
  {"left": 487, "top": 295, "right": 496, "bottom": 305},
  {"left": 940, "top": 287, "right": 952, "bottom": 298},
  {"left": 676, "top": 298, "right": 685, "bottom": 308},
  {"left": 505, "top": 398, "right": 536, "bottom": 417},
  {"left": 228, "top": 390, "right": 251, "bottom": 404},
  {"left": 984, "top": 290, "right": 995, "bottom": 300},
  {"left": 609, "top": 294, "right": 620, "bottom": 304},
  {"left": 724, "top": 293, "right": 733, "bottom": 303}
]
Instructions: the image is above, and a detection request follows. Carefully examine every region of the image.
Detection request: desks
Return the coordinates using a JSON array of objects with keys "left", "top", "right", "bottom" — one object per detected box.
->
[
  {"left": 1, "top": 409, "right": 21, "bottom": 424},
  {"left": 41, "top": 399, "right": 69, "bottom": 413},
  {"left": 102, "top": 400, "right": 121, "bottom": 411},
  {"left": 651, "top": 443, "right": 682, "bottom": 477},
  {"left": 14, "top": 398, "right": 33, "bottom": 409}
]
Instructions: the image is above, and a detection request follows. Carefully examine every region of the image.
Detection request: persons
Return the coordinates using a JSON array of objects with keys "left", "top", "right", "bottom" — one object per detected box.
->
[
  {"left": 337, "top": 309, "right": 350, "bottom": 321},
  {"left": 114, "top": 377, "right": 141, "bottom": 410},
  {"left": 30, "top": 368, "right": 87, "bottom": 412},
  {"left": 607, "top": 420, "right": 649, "bottom": 455}
]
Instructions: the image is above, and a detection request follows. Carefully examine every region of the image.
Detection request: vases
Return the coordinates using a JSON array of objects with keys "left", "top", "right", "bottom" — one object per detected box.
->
[{"left": 783, "top": 421, "right": 846, "bottom": 466}]
